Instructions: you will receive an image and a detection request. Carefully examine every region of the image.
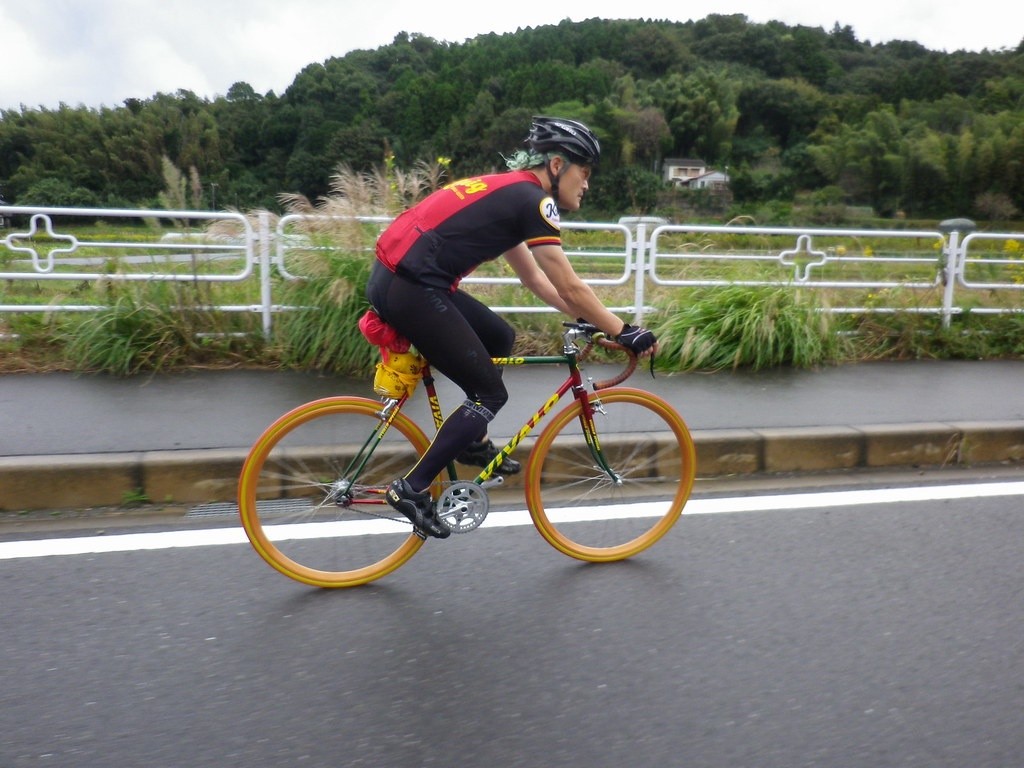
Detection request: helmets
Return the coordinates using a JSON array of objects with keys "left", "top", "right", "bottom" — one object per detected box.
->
[{"left": 526, "top": 116, "right": 601, "bottom": 166}]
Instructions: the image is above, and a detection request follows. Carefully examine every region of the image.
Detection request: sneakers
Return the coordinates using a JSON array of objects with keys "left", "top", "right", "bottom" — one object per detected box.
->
[
  {"left": 386, "top": 478, "right": 450, "bottom": 539},
  {"left": 455, "top": 439, "right": 521, "bottom": 474}
]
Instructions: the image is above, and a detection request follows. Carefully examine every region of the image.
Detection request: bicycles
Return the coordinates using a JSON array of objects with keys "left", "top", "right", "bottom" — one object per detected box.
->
[{"left": 236, "top": 305, "right": 703, "bottom": 590}]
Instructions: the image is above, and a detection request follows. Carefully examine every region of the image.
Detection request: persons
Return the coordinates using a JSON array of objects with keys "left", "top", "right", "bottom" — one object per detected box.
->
[{"left": 368, "top": 115, "right": 659, "bottom": 539}]
